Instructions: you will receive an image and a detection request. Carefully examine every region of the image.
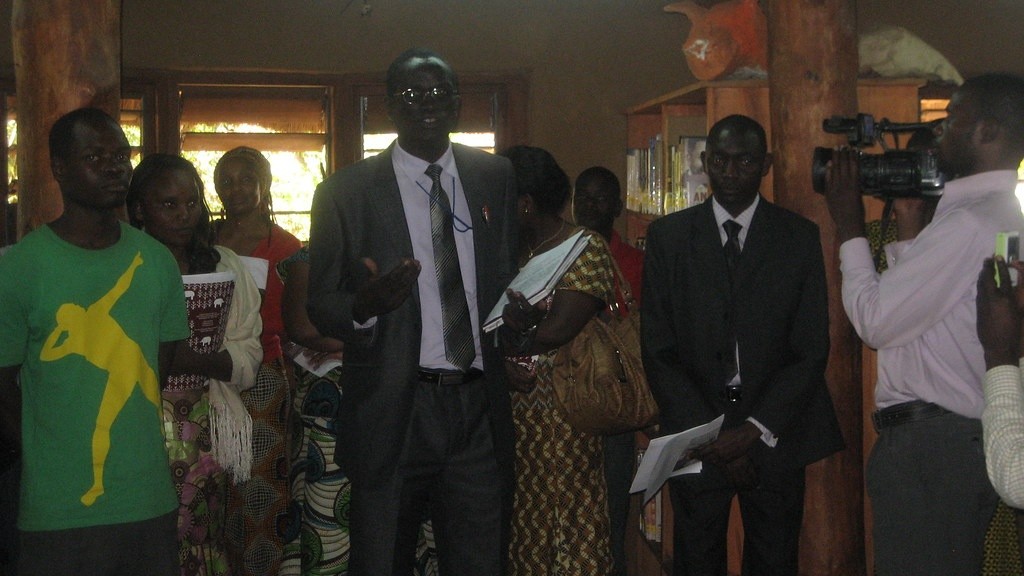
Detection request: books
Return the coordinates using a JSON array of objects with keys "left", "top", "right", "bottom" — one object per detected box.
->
[
  {"left": 483, "top": 229, "right": 592, "bottom": 332},
  {"left": 162, "top": 270, "right": 237, "bottom": 392}
]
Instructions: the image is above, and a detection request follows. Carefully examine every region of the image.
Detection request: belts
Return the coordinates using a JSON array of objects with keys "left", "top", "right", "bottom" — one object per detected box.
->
[
  {"left": 871, "top": 400, "right": 953, "bottom": 434},
  {"left": 724, "top": 386, "right": 747, "bottom": 402},
  {"left": 416, "top": 369, "right": 484, "bottom": 387}
]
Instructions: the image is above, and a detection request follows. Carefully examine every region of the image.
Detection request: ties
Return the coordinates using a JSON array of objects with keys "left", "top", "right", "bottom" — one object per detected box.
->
[
  {"left": 423, "top": 164, "right": 477, "bottom": 374},
  {"left": 723, "top": 219, "right": 753, "bottom": 384}
]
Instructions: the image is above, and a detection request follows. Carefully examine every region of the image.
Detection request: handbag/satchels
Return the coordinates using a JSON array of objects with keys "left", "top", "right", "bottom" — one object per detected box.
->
[{"left": 551, "top": 231, "right": 660, "bottom": 435}]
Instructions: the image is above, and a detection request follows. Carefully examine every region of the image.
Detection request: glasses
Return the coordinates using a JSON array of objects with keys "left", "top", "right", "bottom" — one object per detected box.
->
[{"left": 388, "top": 85, "right": 460, "bottom": 107}]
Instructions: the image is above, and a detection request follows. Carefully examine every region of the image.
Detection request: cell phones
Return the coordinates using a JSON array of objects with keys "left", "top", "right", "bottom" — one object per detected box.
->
[{"left": 993, "top": 231, "right": 1019, "bottom": 287}]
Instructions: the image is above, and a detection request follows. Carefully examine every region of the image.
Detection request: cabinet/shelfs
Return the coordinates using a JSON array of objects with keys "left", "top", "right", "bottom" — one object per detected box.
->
[
  {"left": 858, "top": 77, "right": 928, "bottom": 576},
  {"left": 621, "top": 77, "right": 774, "bottom": 576}
]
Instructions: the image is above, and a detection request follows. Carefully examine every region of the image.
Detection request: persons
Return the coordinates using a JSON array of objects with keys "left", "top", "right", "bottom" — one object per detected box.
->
[
  {"left": 208, "top": 145, "right": 338, "bottom": 576},
  {"left": 306, "top": 49, "right": 548, "bottom": 576},
  {"left": 573, "top": 166, "right": 645, "bottom": 316},
  {"left": 127, "top": 154, "right": 265, "bottom": 576},
  {"left": 0, "top": 107, "right": 192, "bottom": 576},
  {"left": 496, "top": 145, "right": 614, "bottom": 576},
  {"left": 824, "top": 73, "right": 1024, "bottom": 576},
  {"left": 975, "top": 254, "right": 1024, "bottom": 508},
  {"left": 640, "top": 113, "right": 847, "bottom": 576}
]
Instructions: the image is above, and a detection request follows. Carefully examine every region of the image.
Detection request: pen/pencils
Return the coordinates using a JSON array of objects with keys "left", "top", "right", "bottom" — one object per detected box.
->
[{"left": 482, "top": 205, "right": 490, "bottom": 223}]
